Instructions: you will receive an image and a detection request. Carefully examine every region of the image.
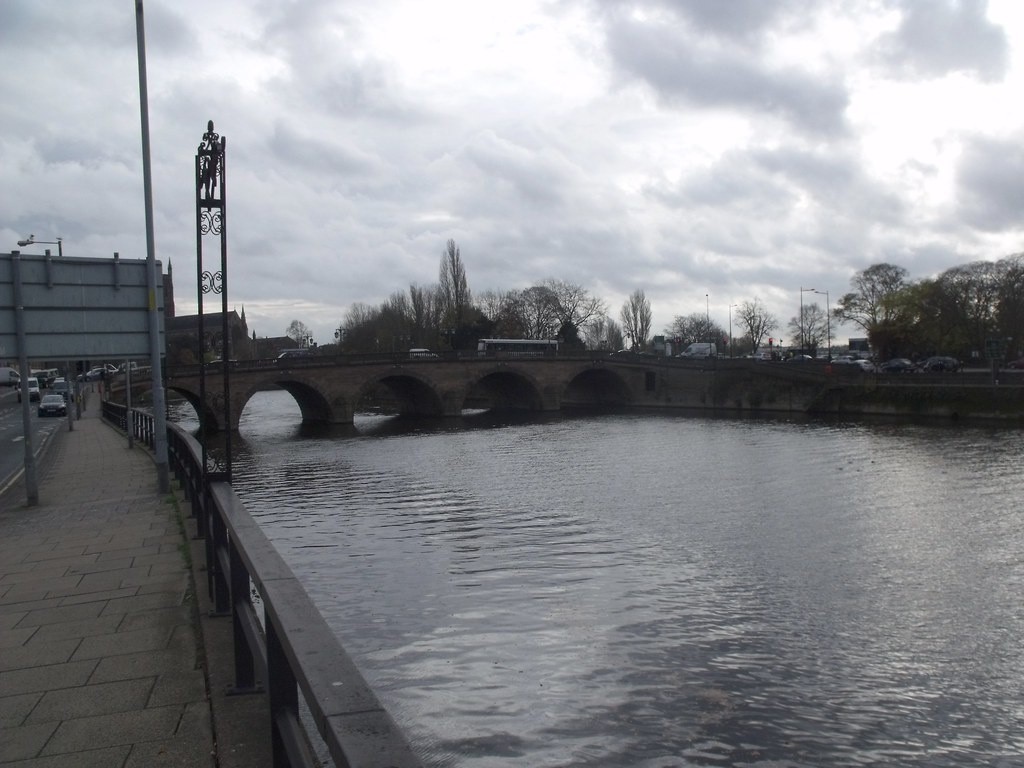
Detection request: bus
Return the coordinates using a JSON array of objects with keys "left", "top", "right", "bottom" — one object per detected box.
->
[{"left": 477, "top": 338, "right": 558, "bottom": 357}]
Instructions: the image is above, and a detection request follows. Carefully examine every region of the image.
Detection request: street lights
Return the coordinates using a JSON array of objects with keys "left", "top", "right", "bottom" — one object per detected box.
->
[
  {"left": 15, "top": 239, "right": 62, "bottom": 257},
  {"left": 814, "top": 291, "right": 831, "bottom": 360},
  {"left": 728, "top": 304, "right": 737, "bottom": 358},
  {"left": 800, "top": 287, "right": 815, "bottom": 359}
]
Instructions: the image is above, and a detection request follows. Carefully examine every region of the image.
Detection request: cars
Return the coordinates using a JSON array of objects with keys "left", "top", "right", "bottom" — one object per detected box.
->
[
  {"left": 0, "top": 361, "right": 137, "bottom": 403},
  {"left": 610, "top": 342, "right": 1023, "bottom": 377},
  {"left": 36, "top": 394, "right": 67, "bottom": 418}
]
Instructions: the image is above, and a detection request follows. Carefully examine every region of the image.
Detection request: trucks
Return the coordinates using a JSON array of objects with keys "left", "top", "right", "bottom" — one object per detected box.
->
[{"left": 409, "top": 349, "right": 438, "bottom": 359}]
